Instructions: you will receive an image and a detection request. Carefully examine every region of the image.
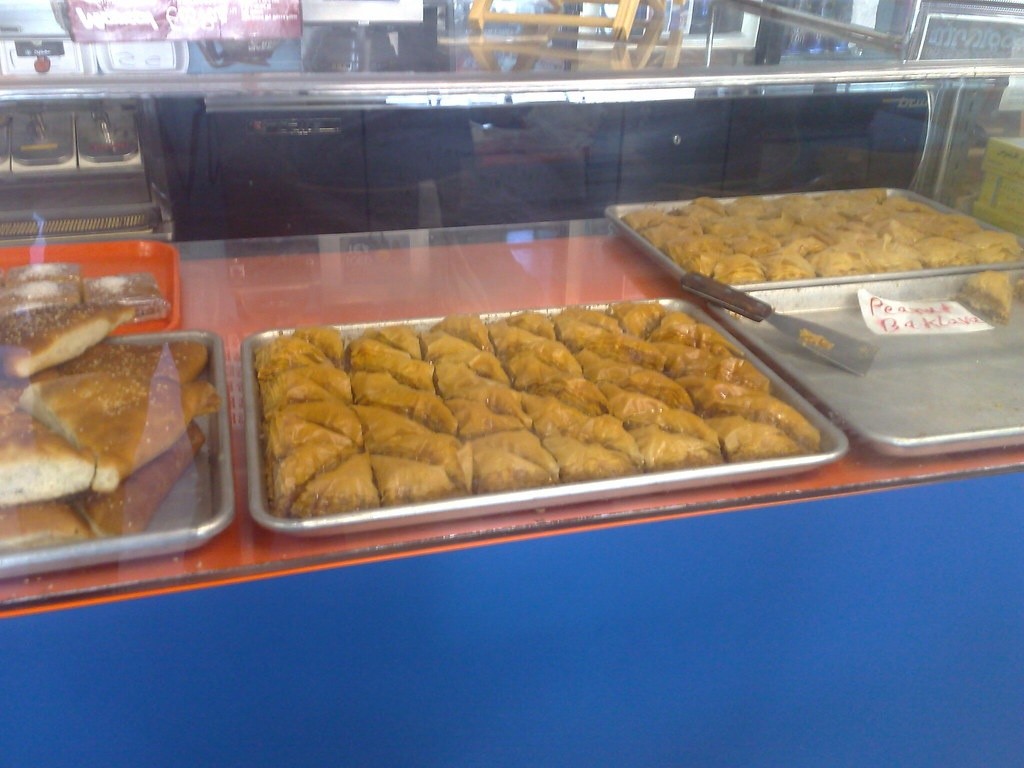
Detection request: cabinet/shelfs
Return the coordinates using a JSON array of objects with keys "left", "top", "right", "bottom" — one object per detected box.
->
[
  {"left": 209, "top": 104, "right": 625, "bottom": 238},
  {"left": 618, "top": 89, "right": 1002, "bottom": 205}
]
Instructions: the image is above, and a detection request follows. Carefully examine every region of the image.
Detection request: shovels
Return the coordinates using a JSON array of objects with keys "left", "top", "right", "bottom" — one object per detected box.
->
[{"left": 681, "top": 269, "right": 880, "bottom": 377}]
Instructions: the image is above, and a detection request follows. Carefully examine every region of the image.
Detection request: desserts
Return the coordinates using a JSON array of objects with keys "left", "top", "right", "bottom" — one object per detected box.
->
[
  {"left": 618, "top": 184, "right": 1024, "bottom": 324},
  {"left": 252, "top": 299, "right": 822, "bottom": 518}
]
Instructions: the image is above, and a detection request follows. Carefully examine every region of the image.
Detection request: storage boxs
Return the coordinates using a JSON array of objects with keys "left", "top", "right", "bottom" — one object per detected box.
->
[{"left": 972, "top": 136, "right": 1024, "bottom": 238}]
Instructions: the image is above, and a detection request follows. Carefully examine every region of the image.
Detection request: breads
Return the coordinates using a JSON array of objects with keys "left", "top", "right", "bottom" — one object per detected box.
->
[{"left": 0, "top": 260, "right": 224, "bottom": 554}]
